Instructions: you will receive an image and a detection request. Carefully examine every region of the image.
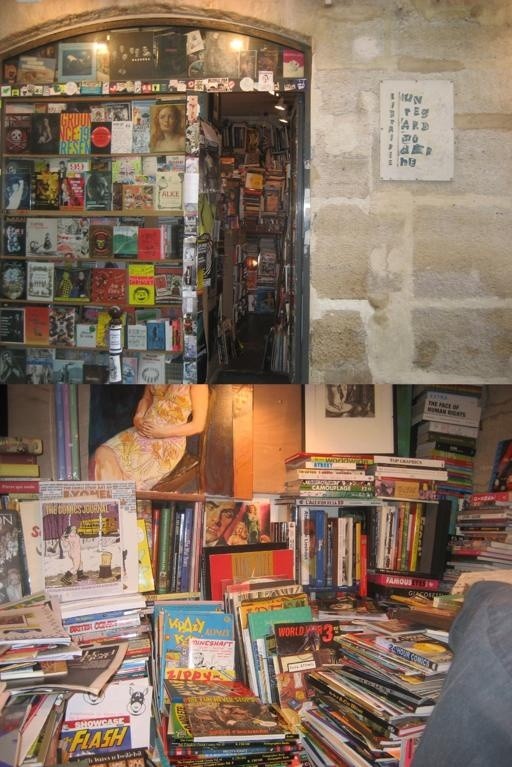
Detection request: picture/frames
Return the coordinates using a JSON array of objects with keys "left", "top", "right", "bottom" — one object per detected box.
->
[{"left": 300, "top": 386, "right": 399, "bottom": 456}]
